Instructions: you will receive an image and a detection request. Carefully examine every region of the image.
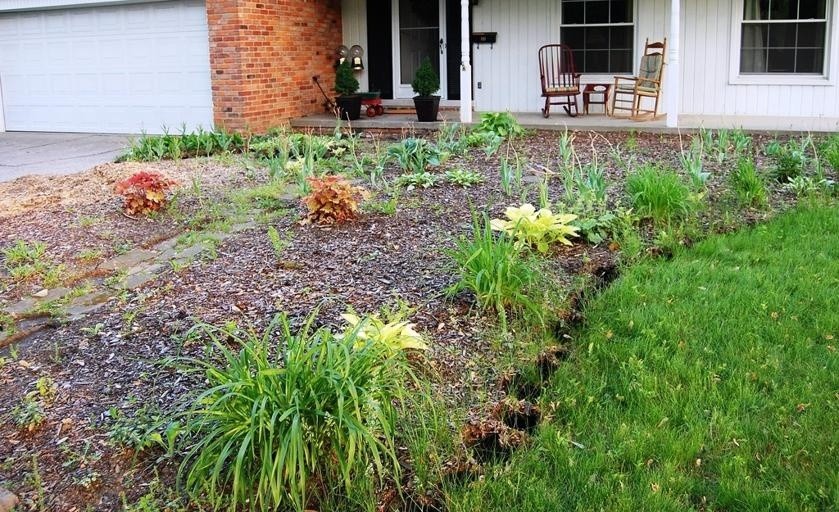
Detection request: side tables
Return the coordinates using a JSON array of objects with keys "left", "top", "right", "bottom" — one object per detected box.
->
[{"left": 582, "top": 81, "right": 612, "bottom": 116}]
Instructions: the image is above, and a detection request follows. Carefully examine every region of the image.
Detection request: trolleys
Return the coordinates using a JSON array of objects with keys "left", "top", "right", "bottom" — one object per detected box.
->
[{"left": 311, "top": 74, "right": 384, "bottom": 118}]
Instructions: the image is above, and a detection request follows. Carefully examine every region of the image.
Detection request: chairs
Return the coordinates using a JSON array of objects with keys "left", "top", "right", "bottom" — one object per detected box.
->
[
  {"left": 608, "top": 37, "right": 668, "bottom": 121},
  {"left": 537, "top": 43, "right": 583, "bottom": 117}
]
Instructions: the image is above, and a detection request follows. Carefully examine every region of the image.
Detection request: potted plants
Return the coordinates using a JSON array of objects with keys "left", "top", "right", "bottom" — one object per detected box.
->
[
  {"left": 410, "top": 56, "right": 444, "bottom": 122},
  {"left": 330, "top": 57, "right": 363, "bottom": 121}
]
